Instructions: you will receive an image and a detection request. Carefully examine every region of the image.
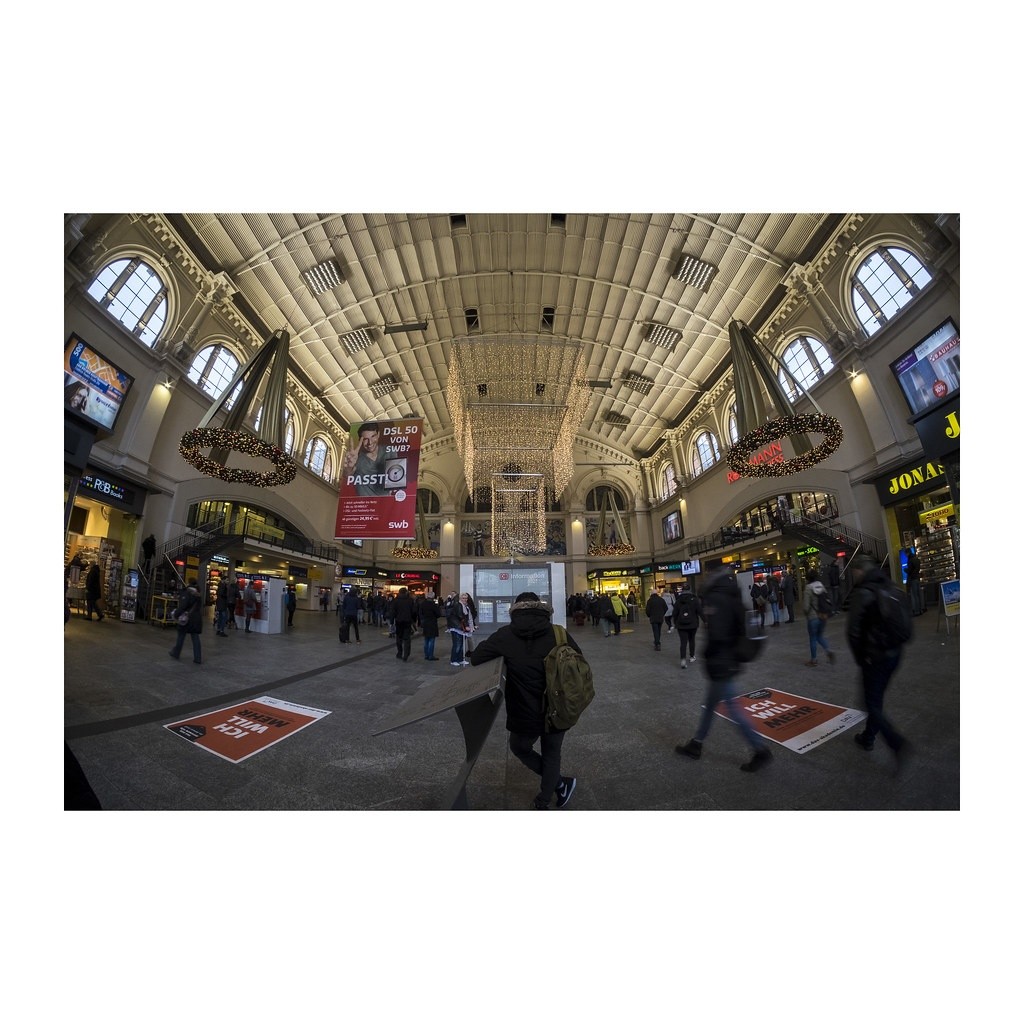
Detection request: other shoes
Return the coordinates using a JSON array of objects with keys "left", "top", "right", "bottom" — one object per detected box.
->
[
  {"left": 217, "top": 620, "right": 293, "bottom": 637},
  {"left": 169, "top": 651, "right": 178, "bottom": 660},
  {"left": 855, "top": 733, "right": 875, "bottom": 750},
  {"left": 805, "top": 661, "right": 817, "bottom": 667},
  {"left": 681, "top": 661, "right": 687, "bottom": 669},
  {"left": 655, "top": 644, "right": 661, "bottom": 651},
  {"left": 690, "top": 656, "right": 696, "bottom": 662},
  {"left": 342, "top": 631, "right": 475, "bottom": 666},
  {"left": 97, "top": 615, "right": 104, "bottom": 621},
  {"left": 827, "top": 651, "right": 837, "bottom": 664},
  {"left": 786, "top": 617, "right": 794, "bottom": 623},
  {"left": 892, "top": 740, "right": 914, "bottom": 776},
  {"left": 84, "top": 616, "right": 92, "bottom": 621}
]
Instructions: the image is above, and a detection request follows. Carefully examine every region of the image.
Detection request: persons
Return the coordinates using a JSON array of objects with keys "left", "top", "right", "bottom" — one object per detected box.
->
[
  {"left": 669, "top": 589, "right": 675, "bottom": 600},
  {"left": 470, "top": 592, "right": 582, "bottom": 809},
  {"left": 750, "top": 576, "right": 769, "bottom": 629},
  {"left": 846, "top": 555, "right": 918, "bottom": 778},
  {"left": 781, "top": 569, "right": 795, "bottom": 623},
  {"left": 343, "top": 424, "right": 397, "bottom": 495},
  {"left": 568, "top": 593, "right": 617, "bottom": 637},
  {"left": 903, "top": 547, "right": 927, "bottom": 616},
  {"left": 611, "top": 593, "right": 628, "bottom": 632},
  {"left": 207, "top": 576, "right": 297, "bottom": 637},
  {"left": 323, "top": 586, "right": 441, "bottom": 661},
  {"left": 627, "top": 591, "right": 637, "bottom": 605},
  {"left": 673, "top": 584, "right": 708, "bottom": 667},
  {"left": 828, "top": 559, "right": 841, "bottom": 615},
  {"left": 64, "top": 380, "right": 90, "bottom": 414},
  {"left": 803, "top": 574, "right": 837, "bottom": 666},
  {"left": 473, "top": 524, "right": 487, "bottom": 557},
  {"left": 766, "top": 575, "right": 780, "bottom": 627},
  {"left": 606, "top": 520, "right": 617, "bottom": 544},
  {"left": 661, "top": 588, "right": 675, "bottom": 633},
  {"left": 646, "top": 591, "right": 668, "bottom": 651},
  {"left": 63, "top": 533, "right": 203, "bottom": 663},
  {"left": 676, "top": 561, "right": 773, "bottom": 772}
]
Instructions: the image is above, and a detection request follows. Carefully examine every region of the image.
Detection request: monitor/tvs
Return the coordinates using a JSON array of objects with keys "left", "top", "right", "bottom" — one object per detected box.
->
[
  {"left": 681, "top": 560, "right": 701, "bottom": 578},
  {"left": 64, "top": 331, "right": 136, "bottom": 433},
  {"left": 662, "top": 510, "right": 683, "bottom": 544},
  {"left": 889, "top": 315, "right": 960, "bottom": 414}
]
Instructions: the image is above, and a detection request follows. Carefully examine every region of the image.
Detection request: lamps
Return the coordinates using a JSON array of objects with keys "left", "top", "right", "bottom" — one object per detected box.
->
[
  {"left": 166, "top": 376, "right": 172, "bottom": 388},
  {"left": 575, "top": 515, "right": 578, "bottom": 520},
  {"left": 848, "top": 364, "right": 858, "bottom": 378},
  {"left": 447, "top": 518, "right": 450, "bottom": 523}
]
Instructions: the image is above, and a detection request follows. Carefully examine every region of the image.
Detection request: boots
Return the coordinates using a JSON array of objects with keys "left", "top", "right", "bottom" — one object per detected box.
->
[
  {"left": 675, "top": 738, "right": 702, "bottom": 760},
  {"left": 740, "top": 745, "right": 774, "bottom": 773}
]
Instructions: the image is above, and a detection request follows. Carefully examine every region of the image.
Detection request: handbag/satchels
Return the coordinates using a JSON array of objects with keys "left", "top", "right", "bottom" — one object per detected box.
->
[
  {"left": 172, "top": 609, "right": 188, "bottom": 625},
  {"left": 768, "top": 591, "right": 777, "bottom": 603},
  {"left": 606, "top": 609, "right": 617, "bottom": 622},
  {"left": 755, "top": 596, "right": 765, "bottom": 606}
]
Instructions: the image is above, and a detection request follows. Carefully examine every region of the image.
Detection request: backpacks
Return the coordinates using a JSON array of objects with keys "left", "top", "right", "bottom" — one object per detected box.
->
[
  {"left": 542, "top": 626, "right": 596, "bottom": 731},
  {"left": 859, "top": 583, "right": 912, "bottom": 645},
  {"left": 677, "top": 594, "right": 695, "bottom": 625},
  {"left": 810, "top": 592, "right": 834, "bottom": 619}
]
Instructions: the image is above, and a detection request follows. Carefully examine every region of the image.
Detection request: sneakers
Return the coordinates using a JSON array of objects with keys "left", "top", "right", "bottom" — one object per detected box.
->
[{"left": 556, "top": 777, "right": 577, "bottom": 807}]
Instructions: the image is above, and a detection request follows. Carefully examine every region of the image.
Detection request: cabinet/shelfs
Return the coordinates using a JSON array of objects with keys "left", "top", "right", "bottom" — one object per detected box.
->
[{"left": 914, "top": 527, "right": 959, "bottom": 584}]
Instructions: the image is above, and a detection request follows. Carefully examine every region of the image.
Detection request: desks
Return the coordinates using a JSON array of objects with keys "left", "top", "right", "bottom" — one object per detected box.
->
[{"left": 149, "top": 595, "right": 180, "bottom": 630}]
